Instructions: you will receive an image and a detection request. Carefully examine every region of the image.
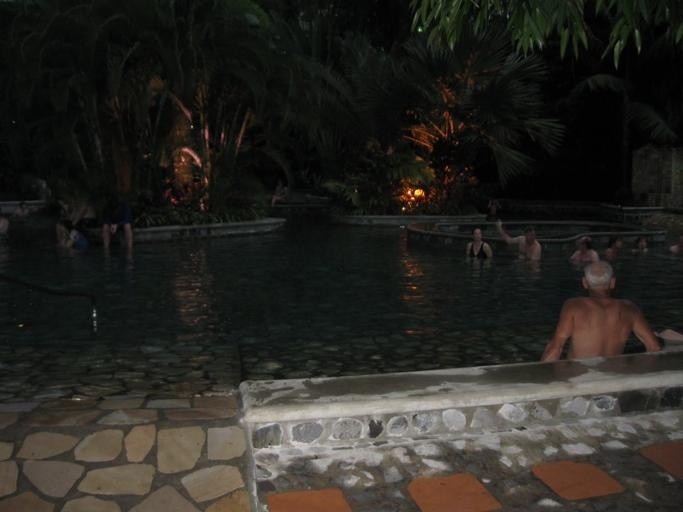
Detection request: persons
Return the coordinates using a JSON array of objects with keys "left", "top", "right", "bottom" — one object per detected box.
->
[
  {"left": 0, "top": 176, "right": 135, "bottom": 260},
  {"left": 464, "top": 218, "right": 683, "bottom": 265},
  {"left": 540, "top": 261, "right": 661, "bottom": 364}
]
